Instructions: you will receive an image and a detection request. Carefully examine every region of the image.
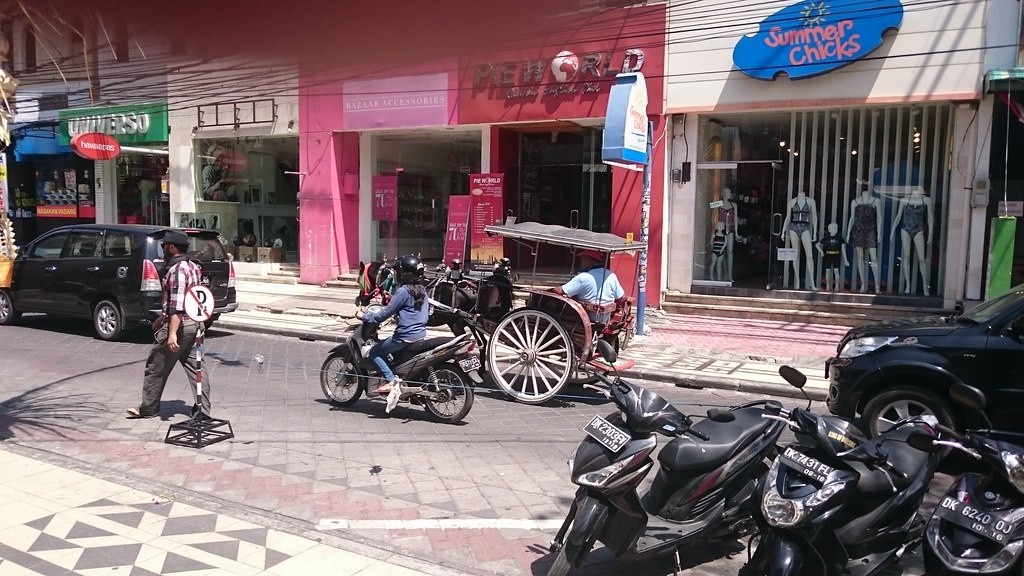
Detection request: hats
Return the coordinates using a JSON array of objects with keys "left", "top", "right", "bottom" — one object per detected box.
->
[
  {"left": 576, "top": 250, "right": 605, "bottom": 261},
  {"left": 160, "top": 228, "right": 190, "bottom": 245}
]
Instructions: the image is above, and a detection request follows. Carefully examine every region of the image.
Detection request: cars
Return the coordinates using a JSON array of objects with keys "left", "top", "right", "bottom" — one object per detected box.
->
[
  {"left": 0, "top": 223, "right": 240, "bottom": 343},
  {"left": 823, "top": 285, "right": 1024, "bottom": 472}
]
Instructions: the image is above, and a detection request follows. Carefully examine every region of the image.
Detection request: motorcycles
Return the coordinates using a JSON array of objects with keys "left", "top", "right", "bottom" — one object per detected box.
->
[{"left": 319, "top": 303, "right": 484, "bottom": 425}]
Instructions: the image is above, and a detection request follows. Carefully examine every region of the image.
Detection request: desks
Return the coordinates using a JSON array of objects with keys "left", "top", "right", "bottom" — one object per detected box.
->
[{"left": 239, "top": 202, "right": 299, "bottom": 247}]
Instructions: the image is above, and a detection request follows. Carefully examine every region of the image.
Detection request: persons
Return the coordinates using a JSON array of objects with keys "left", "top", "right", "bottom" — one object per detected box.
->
[
  {"left": 709, "top": 222, "right": 726, "bottom": 281},
  {"left": 126, "top": 232, "right": 210, "bottom": 421},
  {"left": 548, "top": 250, "right": 635, "bottom": 324},
  {"left": 815, "top": 223, "right": 849, "bottom": 291},
  {"left": 711, "top": 188, "right": 741, "bottom": 280},
  {"left": 203, "top": 145, "right": 235, "bottom": 200},
  {"left": 889, "top": 189, "right": 932, "bottom": 297},
  {"left": 781, "top": 192, "right": 817, "bottom": 291},
  {"left": 846, "top": 191, "right": 882, "bottom": 293},
  {"left": 358, "top": 256, "right": 428, "bottom": 392}
]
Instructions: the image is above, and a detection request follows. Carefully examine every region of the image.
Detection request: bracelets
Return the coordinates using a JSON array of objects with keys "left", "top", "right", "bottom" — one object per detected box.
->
[{"left": 550, "top": 288, "right": 554, "bottom": 292}]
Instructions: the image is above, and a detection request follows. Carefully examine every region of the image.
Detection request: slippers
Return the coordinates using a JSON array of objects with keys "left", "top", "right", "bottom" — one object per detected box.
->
[{"left": 127, "top": 406, "right": 159, "bottom": 417}]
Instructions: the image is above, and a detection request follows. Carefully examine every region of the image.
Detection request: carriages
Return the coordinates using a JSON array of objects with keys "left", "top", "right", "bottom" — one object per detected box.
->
[{"left": 353, "top": 221, "right": 649, "bottom": 405}]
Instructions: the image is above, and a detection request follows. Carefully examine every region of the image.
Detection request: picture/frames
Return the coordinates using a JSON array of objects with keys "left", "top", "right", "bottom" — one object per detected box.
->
[{"left": 250, "top": 186, "right": 262, "bottom": 205}]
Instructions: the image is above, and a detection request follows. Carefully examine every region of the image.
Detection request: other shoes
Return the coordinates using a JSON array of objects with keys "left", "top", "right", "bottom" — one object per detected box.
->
[{"left": 372, "top": 384, "right": 393, "bottom": 393}]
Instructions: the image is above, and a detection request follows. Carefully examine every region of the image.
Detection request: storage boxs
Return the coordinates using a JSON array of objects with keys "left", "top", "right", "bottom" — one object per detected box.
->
[
  {"left": 257, "top": 248, "right": 281, "bottom": 263},
  {"left": 239, "top": 246, "right": 257, "bottom": 262}
]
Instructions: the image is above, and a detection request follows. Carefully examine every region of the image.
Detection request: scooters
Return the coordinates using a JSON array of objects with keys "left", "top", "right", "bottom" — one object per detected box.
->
[
  {"left": 742, "top": 365, "right": 941, "bottom": 576},
  {"left": 546, "top": 337, "right": 791, "bottom": 576},
  {"left": 920, "top": 384, "right": 1024, "bottom": 576}
]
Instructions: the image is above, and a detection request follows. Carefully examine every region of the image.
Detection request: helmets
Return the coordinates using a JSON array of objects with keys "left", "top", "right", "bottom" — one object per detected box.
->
[{"left": 392, "top": 255, "right": 425, "bottom": 285}]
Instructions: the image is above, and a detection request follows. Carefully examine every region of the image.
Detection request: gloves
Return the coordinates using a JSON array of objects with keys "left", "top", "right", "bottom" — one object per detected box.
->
[{"left": 354, "top": 310, "right": 365, "bottom": 320}]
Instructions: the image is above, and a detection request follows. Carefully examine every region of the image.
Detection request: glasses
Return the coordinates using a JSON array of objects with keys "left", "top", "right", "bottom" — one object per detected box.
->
[{"left": 160, "top": 243, "right": 177, "bottom": 249}]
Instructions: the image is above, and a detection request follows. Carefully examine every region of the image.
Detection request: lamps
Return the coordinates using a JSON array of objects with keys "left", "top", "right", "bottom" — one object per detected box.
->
[
  {"left": 232, "top": 125, "right": 240, "bottom": 132},
  {"left": 288, "top": 120, "right": 294, "bottom": 131},
  {"left": 192, "top": 126, "right": 198, "bottom": 137}
]
